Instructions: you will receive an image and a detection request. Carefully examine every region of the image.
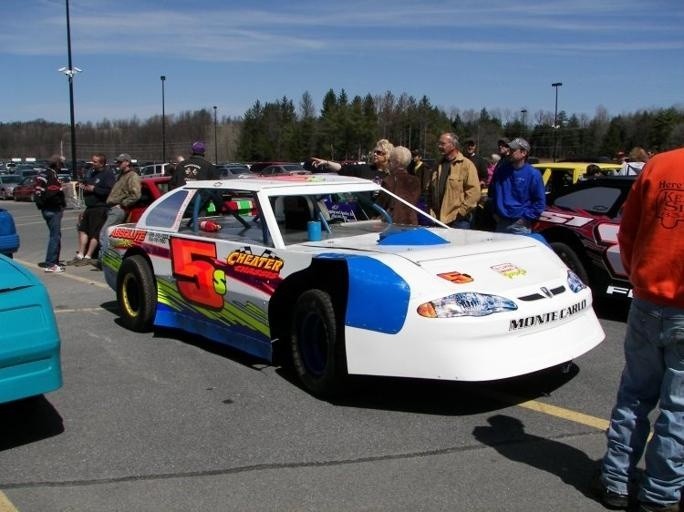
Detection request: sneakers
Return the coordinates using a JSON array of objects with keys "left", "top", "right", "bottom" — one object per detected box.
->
[
  {"left": 41, "top": 256, "right": 101, "bottom": 276},
  {"left": 638, "top": 498, "right": 679, "bottom": 512},
  {"left": 605, "top": 490, "right": 629, "bottom": 508}
]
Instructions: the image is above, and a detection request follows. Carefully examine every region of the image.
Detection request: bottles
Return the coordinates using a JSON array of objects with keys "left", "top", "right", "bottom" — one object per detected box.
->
[
  {"left": 374, "top": 174, "right": 379, "bottom": 196},
  {"left": 198, "top": 220, "right": 221, "bottom": 232}
]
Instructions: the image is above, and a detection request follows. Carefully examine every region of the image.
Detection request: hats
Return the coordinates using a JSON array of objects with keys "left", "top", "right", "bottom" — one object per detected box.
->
[
  {"left": 505, "top": 138, "right": 531, "bottom": 152},
  {"left": 412, "top": 149, "right": 422, "bottom": 156},
  {"left": 192, "top": 142, "right": 205, "bottom": 152},
  {"left": 115, "top": 153, "right": 131, "bottom": 162},
  {"left": 498, "top": 138, "right": 511, "bottom": 144}
]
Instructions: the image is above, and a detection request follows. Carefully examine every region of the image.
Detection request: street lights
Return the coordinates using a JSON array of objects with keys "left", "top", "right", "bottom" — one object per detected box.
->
[
  {"left": 550, "top": 81, "right": 564, "bottom": 126},
  {"left": 159, "top": 74, "right": 168, "bottom": 162},
  {"left": 211, "top": 104, "right": 220, "bottom": 165},
  {"left": 521, "top": 108, "right": 527, "bottom": 125}
]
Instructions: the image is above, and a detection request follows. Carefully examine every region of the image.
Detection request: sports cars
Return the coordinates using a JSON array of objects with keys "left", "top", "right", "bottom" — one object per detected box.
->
[
  {"left": 91, "top": 170, "right": 611, "bottom": 396},
  {"left": 533, "top": 172, "right": 641, "bottom": 303}
]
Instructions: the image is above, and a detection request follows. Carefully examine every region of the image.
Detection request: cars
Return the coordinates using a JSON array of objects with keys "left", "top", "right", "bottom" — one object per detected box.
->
[
  {"left": 0, "top": 250, "right": 65, "bottom": 406},
  {"left": 0, "top": 157, "right": 323, "bottom": 205},
  {"left": 469, "top": 160, "right": 622, "bottom": 212},
  {"left": 0, "top": 209, "right": 24, "bottom": 258}
]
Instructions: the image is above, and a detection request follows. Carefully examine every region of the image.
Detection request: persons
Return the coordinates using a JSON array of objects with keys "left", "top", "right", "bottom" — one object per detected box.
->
[
  {"left": 310, "top": 131, "right": 546, "bottom": 235},
  {"left": 33, "top": 154, "right": 69, "bottom": 274},
  {"left": 88, "top": 152, "right": 142, "bottom": 270},
  {"left": 66, "top": 154, "right": 116, "bottom": 266},
  {"left": 168, "top": 140, "right": 217, "bottom": 188},
  {"left": 591, "top": 146, "right": 684, "bottom": 512},
  {"left": 170, "top": 154, "right": 184, "bottom": 188},
  {"left": 552, "top": 147, "right": 658, "bottom": 195}
]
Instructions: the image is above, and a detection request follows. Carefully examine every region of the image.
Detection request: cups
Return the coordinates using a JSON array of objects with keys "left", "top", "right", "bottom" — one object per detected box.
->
[{"left": 306, "top": 221, "right": 322, "bottom": 241}]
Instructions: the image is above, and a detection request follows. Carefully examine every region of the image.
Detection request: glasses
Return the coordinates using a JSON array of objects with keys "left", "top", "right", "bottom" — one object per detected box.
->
[
  {"left": 468, "top": 144, "right": 474, "bottom": 146},
  {"left": 374, "top": 150, "right": 381, "bottom": 155},
  {"left": 117, "top": 161, "right": 124, "bottom": 165}
]
